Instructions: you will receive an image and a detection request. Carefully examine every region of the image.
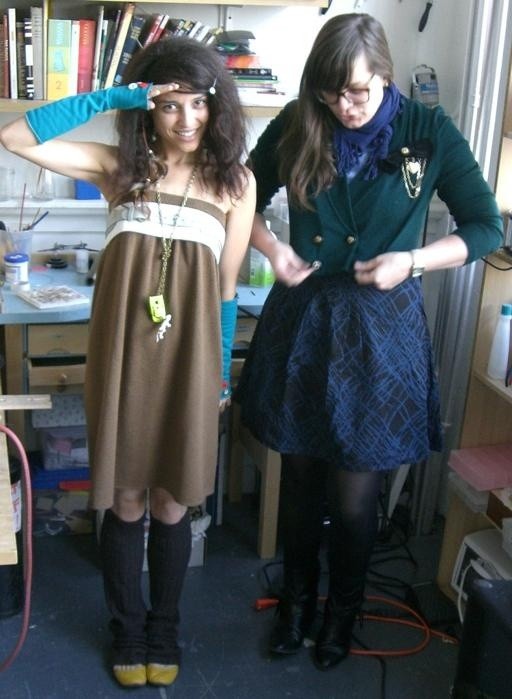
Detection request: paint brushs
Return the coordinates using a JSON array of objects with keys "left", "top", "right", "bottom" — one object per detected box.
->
[{"left": 19, "top": 183, "right": 49, "bottom": 231}]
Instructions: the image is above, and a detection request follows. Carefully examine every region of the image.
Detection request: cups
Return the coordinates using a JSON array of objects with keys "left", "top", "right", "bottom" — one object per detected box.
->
[
  {"left": 1, "top": 167, "right": 15, "bottom": 202},
  {"left": 75, "top": 248, "right": 90, "bottom": 274},
  {"left": 1, "top": 222, "right": 34, "bottom": 276}
]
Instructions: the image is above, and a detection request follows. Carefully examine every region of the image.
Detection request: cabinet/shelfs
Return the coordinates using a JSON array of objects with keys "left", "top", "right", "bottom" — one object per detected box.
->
[
  {"left": 1, "top": 0, "right": 338, "bottom": 120},
  {"left": 426, "top": 33, "right": 512, "bottom": 627}
]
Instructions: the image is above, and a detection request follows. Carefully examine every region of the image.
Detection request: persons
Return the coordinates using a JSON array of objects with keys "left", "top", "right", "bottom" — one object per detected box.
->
[
  {"left": 232, "top": 13, "right": 507, "bottom": 668},
  {"left": 0, "top": 38, "right": 257, "bottom": 688}
]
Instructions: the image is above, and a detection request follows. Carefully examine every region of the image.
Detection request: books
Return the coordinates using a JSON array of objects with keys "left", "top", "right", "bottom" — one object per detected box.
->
[
  {"left": 16, "top": 285, "right": 90, "bottom": 309},
  {"left": 0, "top": 2, "right": 279, "bottom": 102}
]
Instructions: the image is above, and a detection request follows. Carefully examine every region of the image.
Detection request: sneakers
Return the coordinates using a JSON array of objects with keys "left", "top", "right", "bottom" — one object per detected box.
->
[
  {"left": 112, "top": 628, "right": 147, "bottom": 688},
  {"left": 147, "top": 623, "right": 182, "bottom": 685}
]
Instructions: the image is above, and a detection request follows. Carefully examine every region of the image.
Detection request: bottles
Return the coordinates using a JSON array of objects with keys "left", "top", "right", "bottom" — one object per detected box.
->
[
  {"left": 31, "top": 166, "right": 57, "bottom": 200},
  {"left": 249, "top": 219, "right": 275, "bottom": 289},
  {"left": 487, "top": 305, "right": 511, "bottom": 382}
]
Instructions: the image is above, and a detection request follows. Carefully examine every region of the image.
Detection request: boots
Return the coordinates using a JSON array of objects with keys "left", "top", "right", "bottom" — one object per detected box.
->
[
  {"left": 267, "top": 502, "right": 322, "bottom": 654},
  {"left": 311, "top": 517, "right": 379, "bottom": 671}
]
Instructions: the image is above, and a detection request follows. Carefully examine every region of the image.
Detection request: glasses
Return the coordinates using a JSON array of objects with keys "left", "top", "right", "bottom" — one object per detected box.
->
[{"left": 314, "top": 73, "right": 376, "bottom": 106}]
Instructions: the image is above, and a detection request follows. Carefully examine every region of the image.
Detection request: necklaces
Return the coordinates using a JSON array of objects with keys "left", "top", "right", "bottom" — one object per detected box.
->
[{"left": 149, "top": 156, "right": 201, "bottom": 342}]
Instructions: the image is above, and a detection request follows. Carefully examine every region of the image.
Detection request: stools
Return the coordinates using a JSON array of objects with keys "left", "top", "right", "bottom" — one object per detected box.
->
[{"left": 450, "top": 580, "right": 511, "bottom": 698}]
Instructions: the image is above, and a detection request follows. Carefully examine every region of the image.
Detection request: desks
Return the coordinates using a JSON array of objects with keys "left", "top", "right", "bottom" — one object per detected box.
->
[{"left": 1, "top": 248, "right": 285, "bottom": 565}]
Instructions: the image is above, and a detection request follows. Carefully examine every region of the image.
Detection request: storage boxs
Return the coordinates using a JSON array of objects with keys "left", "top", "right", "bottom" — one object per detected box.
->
[
  {"left": 27, "top": 452, "right": 96, "bottom": 537},
  {"left": 30, "top": 393, "right": 92, "bottom": 469}
]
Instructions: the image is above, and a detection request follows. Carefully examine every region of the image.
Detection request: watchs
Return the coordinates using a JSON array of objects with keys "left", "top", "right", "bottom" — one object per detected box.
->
[{"left": 410, "top": 249, "right": 424, "bottom": 278}]
